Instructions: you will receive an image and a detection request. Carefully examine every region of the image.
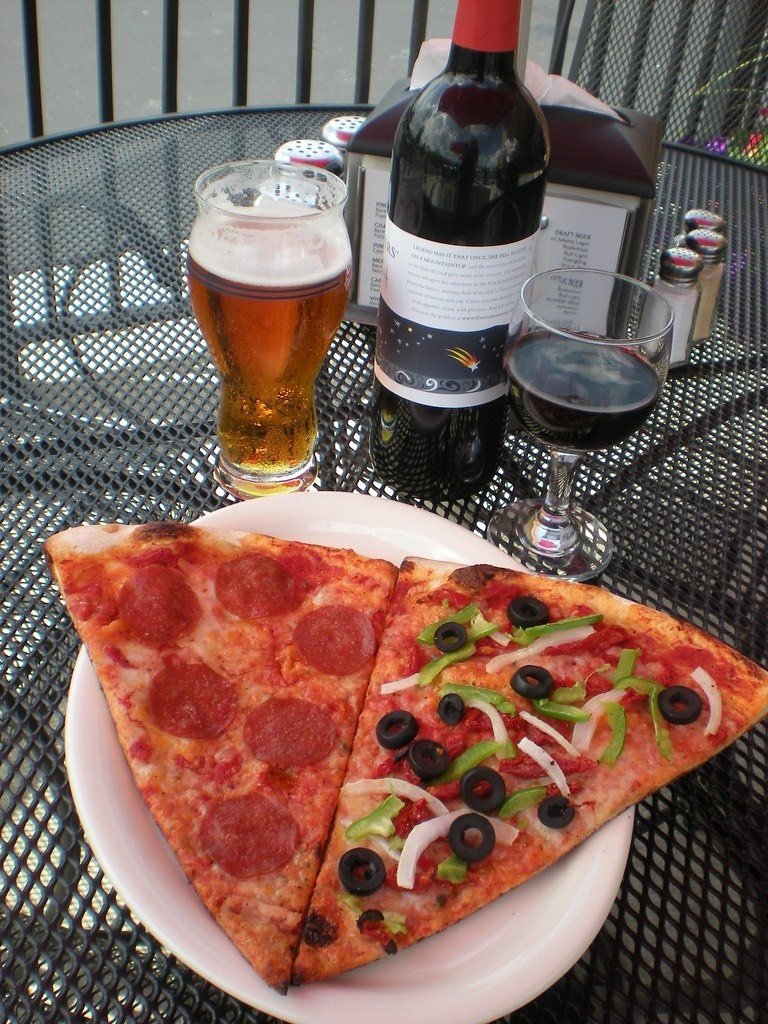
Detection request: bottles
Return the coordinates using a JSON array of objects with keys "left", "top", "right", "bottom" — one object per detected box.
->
[
  {"left": 632, "top": 247, "right": 703, "bottom": 371},
  {"left": 273, "top": 140, "right": 346, "bottom": 187},
  {"left": 671, "top": 208, "right": 727, "bottom": 247},
  {"left": 319, "top": 114, "right": 367, "bottom": 156},
  {"left": 367, "top": 0, "right": 550, "bottom": 504},
  {"left": 678, "top": 229, "right": 729, "bottom": 343}
]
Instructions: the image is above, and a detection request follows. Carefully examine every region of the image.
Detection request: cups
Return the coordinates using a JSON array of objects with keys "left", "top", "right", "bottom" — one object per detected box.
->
[{"left": 184, "top": 158, "right": 353, "bottom": 501}]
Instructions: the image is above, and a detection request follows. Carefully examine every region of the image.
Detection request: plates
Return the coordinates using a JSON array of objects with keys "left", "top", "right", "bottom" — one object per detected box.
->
[{"left": 64, "top": 492, "right": 636, "bottom": 1024}]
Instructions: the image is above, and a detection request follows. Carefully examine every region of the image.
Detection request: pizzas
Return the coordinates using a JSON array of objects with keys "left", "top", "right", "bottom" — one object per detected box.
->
[
  {"left": 45, "top": 520, "right": 400, "bottom": 997},
  {"left": 289, "top": 556, "right": 768, "bottom": 987}
]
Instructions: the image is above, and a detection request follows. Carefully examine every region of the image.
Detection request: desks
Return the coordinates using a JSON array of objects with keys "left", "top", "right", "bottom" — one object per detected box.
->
[{"left": 1, "top": 103, "right": 768, "bottom": 1024}]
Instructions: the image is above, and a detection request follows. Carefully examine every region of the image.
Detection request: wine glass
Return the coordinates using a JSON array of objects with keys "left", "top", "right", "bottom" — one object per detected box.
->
[{"left": 486, "top": 266, "right": 673, "bottom": 583}]
